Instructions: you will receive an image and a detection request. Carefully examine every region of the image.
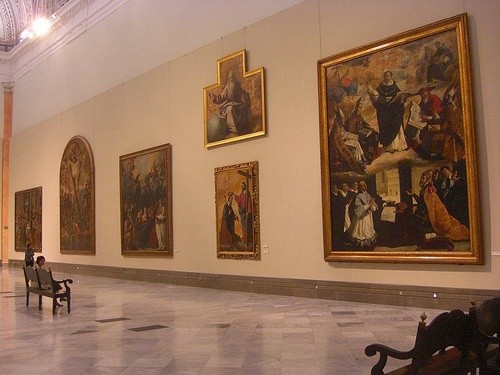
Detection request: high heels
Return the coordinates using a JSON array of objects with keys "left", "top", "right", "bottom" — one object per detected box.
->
[
  {"left": 60, "top": 297, "right": 67, "bottom": 302},
  {"left": 56, "top": 302, "right": 63, "bottom": 307}
]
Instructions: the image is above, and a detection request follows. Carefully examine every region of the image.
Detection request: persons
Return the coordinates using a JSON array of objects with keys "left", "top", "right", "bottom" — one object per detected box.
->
[
  {"left": 25, "top": 243, "right": 34, "bottom": 267},
  {"left": 30, "top": 255, "right": 68, "bottom": 307}
]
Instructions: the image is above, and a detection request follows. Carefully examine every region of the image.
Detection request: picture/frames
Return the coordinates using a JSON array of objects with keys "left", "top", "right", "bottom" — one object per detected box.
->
[
  {"left": 119, "top": 143, "right": 174, "bottom": 257},
  {"left": 317, "top": 13, "right": 485, "bottom": 264},
  {"left": 60, "top": 135, "right": 96, "bottom": 255},
  {"left": 202, "top": 49, "right": 266, "bottom": 149},
  {"left": 214, "top": 161, "right": 261, "bottom": 260},
  {"left": 15, "top": 187, "right": 42, "bottom": 252}
]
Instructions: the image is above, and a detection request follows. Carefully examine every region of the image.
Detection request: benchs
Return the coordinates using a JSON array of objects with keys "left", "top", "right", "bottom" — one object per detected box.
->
[
  {"left": 365, "top": 297, "right": 500, "bottom": 375},
  {"left": 23, "top": 266, "right": 73, "bottom": 315}
]
